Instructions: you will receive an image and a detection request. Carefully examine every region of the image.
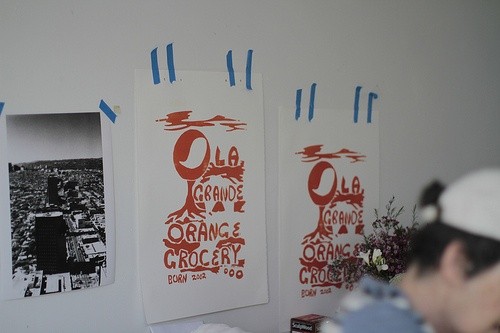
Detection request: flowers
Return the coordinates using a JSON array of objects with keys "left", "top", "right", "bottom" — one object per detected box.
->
[{"left": 331, "top": 196, "right": 423, "bottom": 292}]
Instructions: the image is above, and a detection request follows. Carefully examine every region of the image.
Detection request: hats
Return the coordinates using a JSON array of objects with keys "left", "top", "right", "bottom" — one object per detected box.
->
[{"left": 420, "top": 169, "right": 500, "bottom": 243}]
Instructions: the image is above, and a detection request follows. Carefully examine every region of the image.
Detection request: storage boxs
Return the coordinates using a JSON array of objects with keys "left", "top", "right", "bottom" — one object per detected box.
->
[{"left": 291, "top": 314, "right": 328, "bottom": 333}]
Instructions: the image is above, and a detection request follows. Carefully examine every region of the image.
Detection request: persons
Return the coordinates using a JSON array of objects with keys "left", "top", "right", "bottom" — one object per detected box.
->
[{"left": 318, "top": 165, "right": 500, "bottom": 332}]
